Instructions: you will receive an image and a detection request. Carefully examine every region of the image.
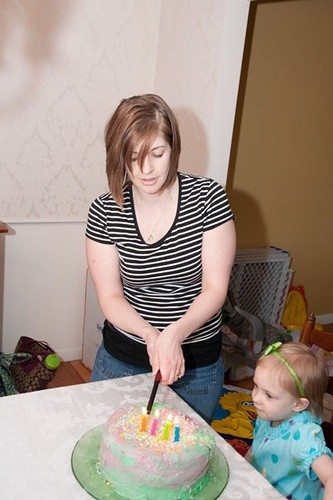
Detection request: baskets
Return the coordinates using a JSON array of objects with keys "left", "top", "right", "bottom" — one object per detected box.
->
[{"left": 9, "top": 336, "right": 57, "bottom": 394}]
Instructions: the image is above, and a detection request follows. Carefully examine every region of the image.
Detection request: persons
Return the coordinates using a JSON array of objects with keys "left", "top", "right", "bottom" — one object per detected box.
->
[
  {"left": 84, "top": 93, "right": 237, "bottom": 425},
  {"left": 243, "top": 340, "right": 333, "bottom": 500}
]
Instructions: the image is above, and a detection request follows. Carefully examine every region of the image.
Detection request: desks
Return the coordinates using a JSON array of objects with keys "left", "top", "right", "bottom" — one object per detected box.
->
[{"left": 0, "top": 373, "right": 288, "bottom": 500}]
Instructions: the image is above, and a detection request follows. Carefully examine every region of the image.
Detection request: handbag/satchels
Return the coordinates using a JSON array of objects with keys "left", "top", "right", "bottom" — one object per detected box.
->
[{"left": 0, "top": 351, "right": 33, "bottom": 397}]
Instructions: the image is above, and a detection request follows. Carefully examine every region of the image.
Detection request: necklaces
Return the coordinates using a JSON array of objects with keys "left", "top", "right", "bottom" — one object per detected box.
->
[{"left": 132, "top": 186, "right": 171, "bottom": 242}]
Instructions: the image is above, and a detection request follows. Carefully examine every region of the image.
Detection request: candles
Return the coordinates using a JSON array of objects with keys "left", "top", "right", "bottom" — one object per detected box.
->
[
  {"left": 149, "top": 409, "right": 160, "bottom": 434},
  {"left": 162, "top": 415, "right": 172, "bottom": 439},
  {"left": 173, "top": 415, "right": 181, "bottom": 441},
  {"left": 140, "top": 406, "right": 147, "bottom": 432}
]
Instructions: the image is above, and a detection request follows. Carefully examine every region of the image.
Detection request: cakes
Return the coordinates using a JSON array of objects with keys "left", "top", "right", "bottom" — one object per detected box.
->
[{"left": 99, "top": 400, "right": 216, "bottom": 500}]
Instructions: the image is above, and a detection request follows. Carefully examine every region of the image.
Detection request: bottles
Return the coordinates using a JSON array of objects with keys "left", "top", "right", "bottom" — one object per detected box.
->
[{"left": 44, "top": 354, "right": 61, "bottom": 370}]
